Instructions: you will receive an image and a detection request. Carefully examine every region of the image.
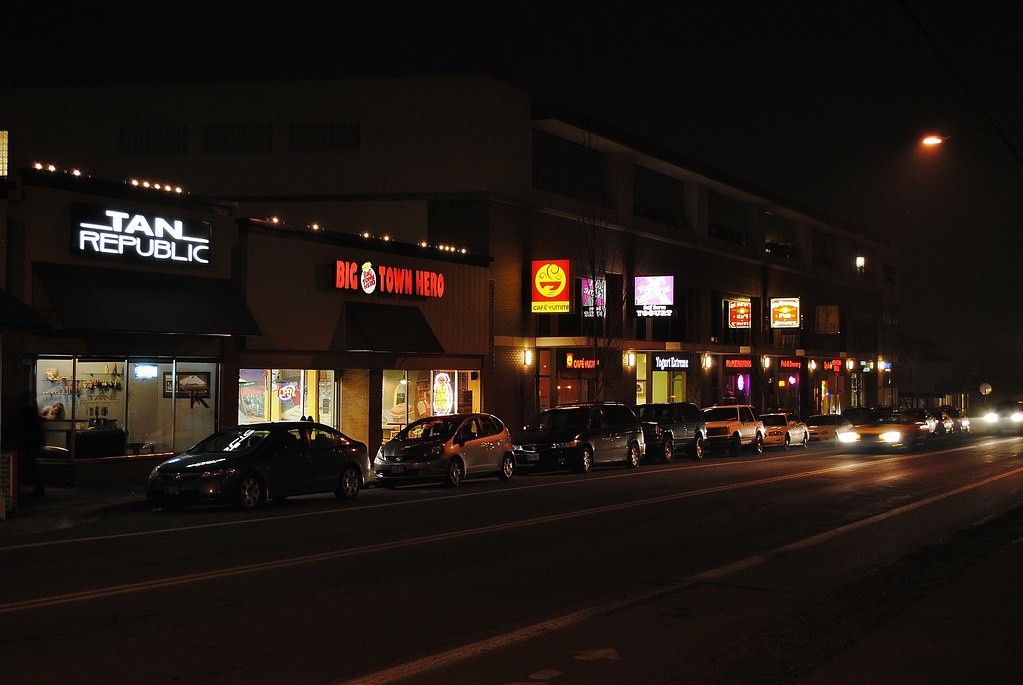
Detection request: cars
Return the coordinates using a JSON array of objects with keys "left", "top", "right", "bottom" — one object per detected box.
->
[
  {"left": 757, "top": 412, "right": 810, "bottom": 452},
  {"left": 933, "top": 412, "right": 954, "bottom": 435},
  {"left": 148, "top": 419, "right": 370, "bottom": 511},
  {"left": 374, "top": 413, "right": 517, "bottom": 487},
  {"left": 24, "top": 447, "right": 86, "bottom": 465},
  {"left": 853, "top": 409, "right": 939, "bottom": 451},
  {"left": 804, "top": 414, "right": 853, "bottom": 447}
]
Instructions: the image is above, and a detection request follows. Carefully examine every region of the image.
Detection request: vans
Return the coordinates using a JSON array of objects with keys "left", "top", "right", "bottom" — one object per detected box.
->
[{"left": 989, "top": 401, "right": 1023, "bottom": 436}]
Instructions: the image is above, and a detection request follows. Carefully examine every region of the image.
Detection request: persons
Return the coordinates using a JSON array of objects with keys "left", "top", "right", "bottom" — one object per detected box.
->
[{"left": 40, "top": 403, "right": 65, "bottom": 420}]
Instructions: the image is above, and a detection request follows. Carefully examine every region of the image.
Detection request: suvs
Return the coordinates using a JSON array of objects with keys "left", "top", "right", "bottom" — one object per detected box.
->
[
  {"left": 513, "top": 400, "right": 645, "bottom": 474},
  {"left": 631, "top": 403, "right": 709, "bottom": 464},
  {"left": 698, "top": 405, "right": 766, "bottom": 459},
  {"left": 843, "top": 406, "right": 896, "bottom": 426}
]
